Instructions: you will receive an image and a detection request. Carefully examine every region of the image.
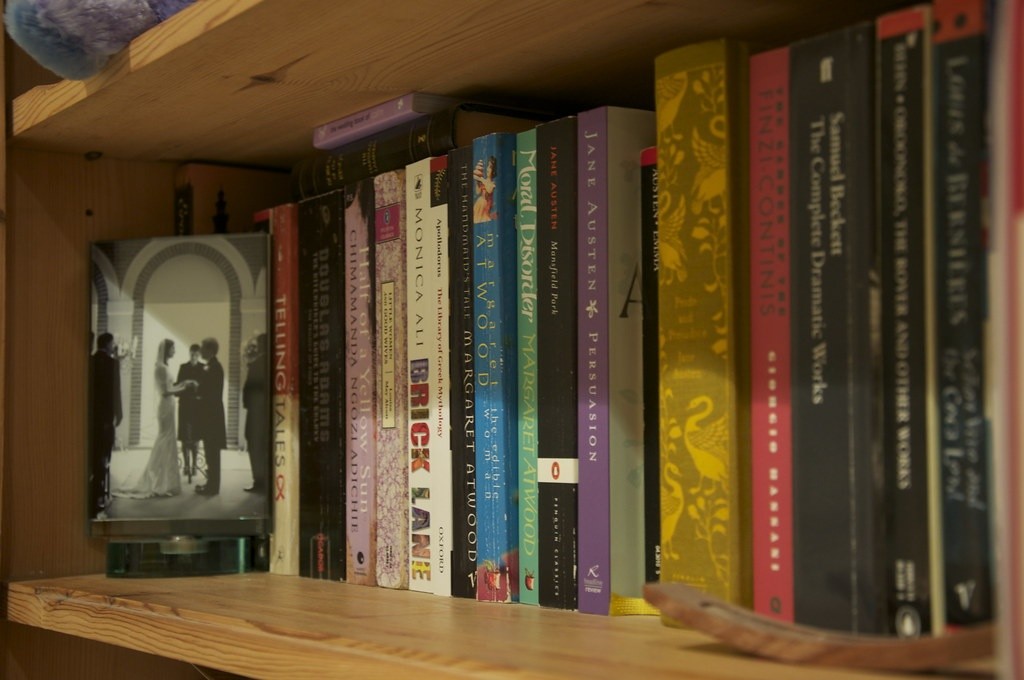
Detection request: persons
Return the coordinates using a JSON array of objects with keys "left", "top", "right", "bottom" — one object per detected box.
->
[
  {"left": 108, "top": 335, "right": 227, "bottom": 499},
  {"left": 90, "top": 332, "right": 120, "bottom": 516},
  {"left": 241, "top": 332, "right": 267, "bottom": 492}
]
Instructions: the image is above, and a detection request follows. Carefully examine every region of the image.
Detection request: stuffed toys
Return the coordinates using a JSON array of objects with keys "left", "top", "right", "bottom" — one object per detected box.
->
[{"left": 4, "top": 0, "right": 194, "bottom": 83}]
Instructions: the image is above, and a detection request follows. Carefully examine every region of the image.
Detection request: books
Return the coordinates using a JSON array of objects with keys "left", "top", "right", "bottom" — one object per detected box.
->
[
  {"left": 91, "top": 231, "right": 270, "bottom": 522},
  {"left": 171, "top": 0, "right": 1024, "bottom": 679}
]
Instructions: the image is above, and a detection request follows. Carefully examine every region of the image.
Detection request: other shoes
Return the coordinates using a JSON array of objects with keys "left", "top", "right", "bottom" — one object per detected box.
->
[
  {"left": 244, "top": 485, "right": 261, "bottom": 494},
  {"left": 195, "top": 485, "right": 219, "bottom": 494}
]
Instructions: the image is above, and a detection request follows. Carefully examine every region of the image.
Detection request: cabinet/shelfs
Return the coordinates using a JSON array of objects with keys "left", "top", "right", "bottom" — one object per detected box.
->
[{"left": 0, "top": 0, "right": 1024, "bottom": 680}]
